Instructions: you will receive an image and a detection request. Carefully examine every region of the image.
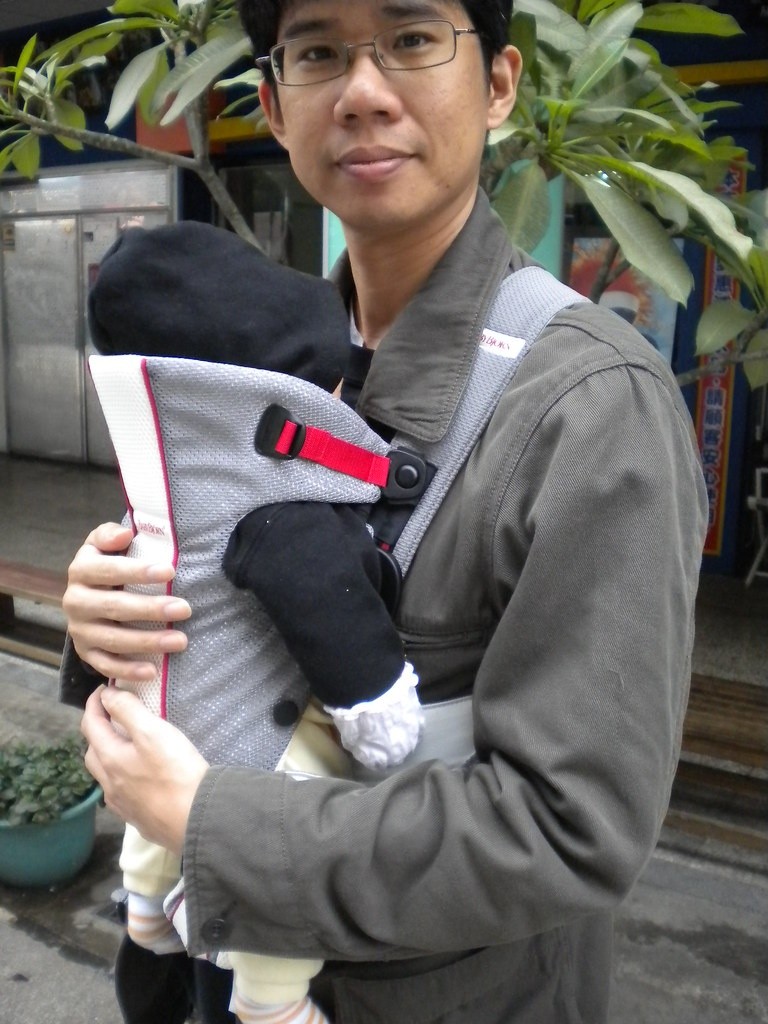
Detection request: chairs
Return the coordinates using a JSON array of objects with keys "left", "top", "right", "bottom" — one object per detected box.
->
[{"left": 743, "top": 466, "right": 768, "bottom": 590}]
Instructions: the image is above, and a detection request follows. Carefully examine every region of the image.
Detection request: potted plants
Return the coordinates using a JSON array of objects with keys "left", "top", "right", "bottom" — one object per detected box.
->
[{"left": 1, "top": 725, "right": 107, "bottom": 890}]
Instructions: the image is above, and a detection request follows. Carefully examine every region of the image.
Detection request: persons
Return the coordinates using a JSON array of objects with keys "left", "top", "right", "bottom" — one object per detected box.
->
[
  {"left": 54, "top": 1, "right": 712, "bottom": 1024},
  {"left": 93, "top": 223, "right": 426, "bottom": 1024}
]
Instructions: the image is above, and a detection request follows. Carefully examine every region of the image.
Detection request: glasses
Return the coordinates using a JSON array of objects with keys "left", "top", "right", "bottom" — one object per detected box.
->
[{"left": 253, "top": 18, "right": 493, "bottom": 87}]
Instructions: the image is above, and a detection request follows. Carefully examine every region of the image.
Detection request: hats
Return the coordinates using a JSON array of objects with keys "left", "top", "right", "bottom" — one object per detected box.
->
[{"left": 86, "top": 217, "right": 355, "bottom": 398}]
